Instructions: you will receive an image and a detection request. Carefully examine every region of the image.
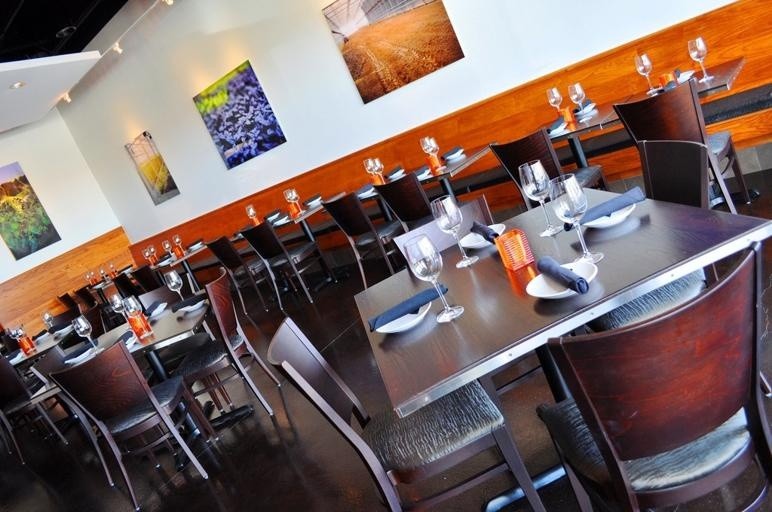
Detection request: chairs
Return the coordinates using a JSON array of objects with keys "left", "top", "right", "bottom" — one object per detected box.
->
[
  {"left": 322, "top": 190, "right": 404, "bottom": 291},
  {"left": 56, "top": 263, "right": 163, "bottom": 328},
  {"left": 240, "top": 219, "right": 338, "bottom": 310},
  {"left": 268, "top": 139, "right": 771, "bottom": 510},
  {"left": 204, "top": 235, "right": 271, "bottom": 316},
  {"left": 30, "top": 267, "right": 282, "bottom": 509},
  {"left": 373, "top": 172, "right": 435, "bottom": 233},
  {"left": 612, "top": 77, "right": 753, "bottom": 215},
  {"left": 1, "top": 301, "right": 107, "bottom": 464},
  {"left": 489, "top": 127, "right": 609, "bottom": 212}
]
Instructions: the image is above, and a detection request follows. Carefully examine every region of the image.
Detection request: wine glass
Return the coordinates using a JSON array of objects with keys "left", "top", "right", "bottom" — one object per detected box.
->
[
  {"left": 402, "top": 233, "right": 466, "bottom": 325},
  {"left": 518, "top": 159, "right": 565, "bottom": 239},
  {"left": 245, "top": 204, "right": 261, "bottom": 227},
  {"left": 83, "top": 262, "right": 118, "bottom": 286},
  {"left": 282, "top": 188, "right": 308, "bottom": 215},
  {"left": 107, "top": 293, "right": 133, "bottom": 332},
  {"left": 71, "top": 314, "right": 104, "bottom": 355},
  {"left": 429, "top": 194, "right": 480, "bottom": 269},
  {"left": 545, "top": 81, "right": 593, "bottom": 123},
  {"left": 40, "top": 311, "right": 56, "bottom": 334},
  {"left": 633, "top": 52, "right": 661, "bottom": 97},
  {"left": 121, "top": 295, "right": 154, "bottom": 339},
  {"left": 7, "top": 317, "right": 31, "bottom": 354},
  {"left": 687, "top": 35, "right": 715, "bottom": 83},
  {"left": 143, "top": 234, "right": 188, "bottom": 270},
  {"left": 361, "top": 156, "right": 387, "bottom": 185},
  {"left": 418, "top": 135, "right": 445, "bottom": 170},
  {"left": 547, "top": 173, "right": 605, "bottom": 265},
  {"left": 164, "top": 270, "right": 185, "bottom": 303}
]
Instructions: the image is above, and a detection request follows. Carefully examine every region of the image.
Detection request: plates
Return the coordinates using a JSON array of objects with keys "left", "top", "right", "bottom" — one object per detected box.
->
[
  {"left": 540, "top": 121, "right": 568, "bottom": 135},
  {"left": 3, "top": 350, "right": 25, "bottom": 364},
  {"left": 61, "top": 347, "right": 93, "bottom": 364},
  {"left": 356, "top": 185, "right": 377, "bottom": 198},
  {"left": 441, "top": 147, "right": 465, "bottom": 161},
  {"left": 525, "top": 262, "right": 598, "bottom": 300},
  {"left": 145, "top": 301, "right": 168, "bottom": 317},
  {"left": 303, "top": 194, "right": 322, "bottom": 209},
  {"left": 572, "top": 102, "right": 597, "bottom": 116},
  {"left": 31, "top": 329, "right": 49, "bottom": 342},
  {"left": 411, "top": 167, "right": 433, "bottom": 182},
  {"left": 187, "top": 239, "right": 204, "bottom": 250},
  {"left": 53, "top": 322, "right": 74, "bottom": 334},
  {"left": 112, "top": 335, "right": 138, "bottom": 349},
  {"left": 91, "top": 280, "right": 106, "bottom": 289},
  {"left": 177, "top": 298, "right": 209, "bottom": 313},
  {"left": 459, "top": 223, "right": 507, "bottom": 250},
  {"left": 264, "top": 210, "right": 291, "bottom": 225},
  {"left": 385, "top": 168, "right": 407, "bottom": 181},
  {"left": 664, "top": 69, "right": 695, "bottom": 85},
  {"left": 577, "top": 202, "right": 637, "bottom": 229},
  {"left": 374, "top": 300, "right": 433, "bottom": 337},
  {"left": 117, "top": 265, "right": 133, "bottom": 274}
]
